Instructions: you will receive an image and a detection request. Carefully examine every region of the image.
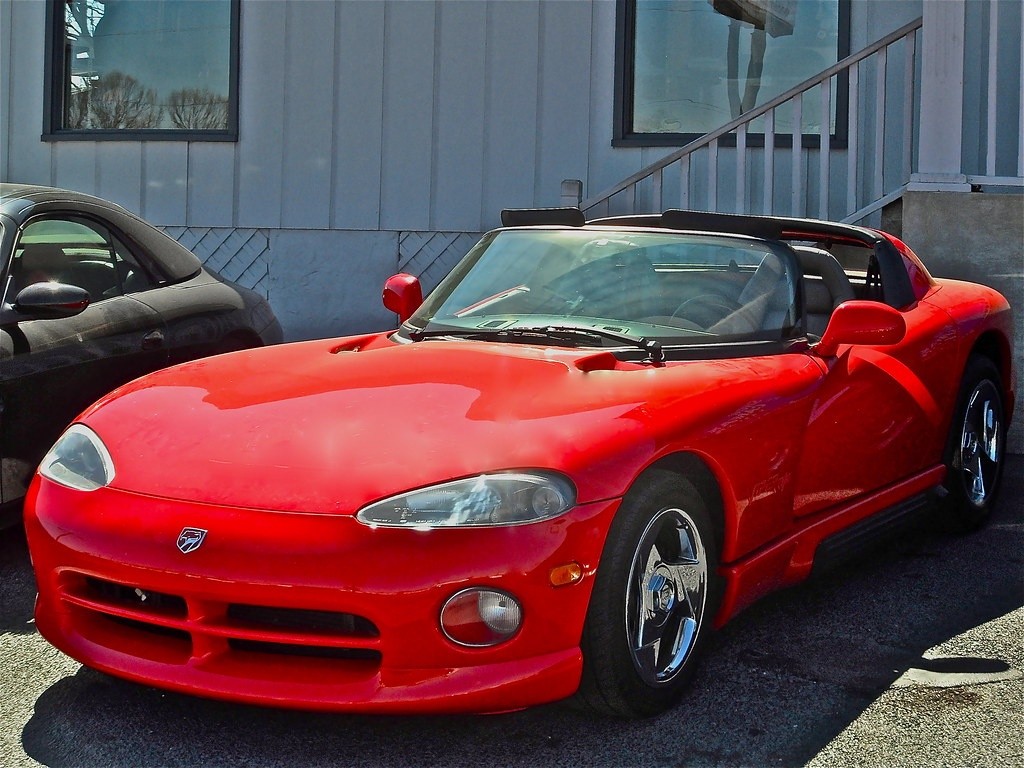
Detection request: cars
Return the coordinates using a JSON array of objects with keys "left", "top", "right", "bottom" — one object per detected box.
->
[{"left": 0, "top": 183, "right": 284, "bottom": 530}]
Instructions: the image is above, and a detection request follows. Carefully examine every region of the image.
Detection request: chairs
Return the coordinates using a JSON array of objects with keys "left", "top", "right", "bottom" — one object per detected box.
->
[
  {"left": 556, "top": 239, "right": 665, "bottom": 323},
  {"left": 735, "top": 247, "right": 855, "bottom": 337},
  {"left": 13, "top": 244, "right": 78, "bottom": 293}
]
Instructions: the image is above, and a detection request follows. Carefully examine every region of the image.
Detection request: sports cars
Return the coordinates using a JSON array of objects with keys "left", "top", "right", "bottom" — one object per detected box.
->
[{"left": 23, "top": 206, "right": 1016, "bottom": 720}]
